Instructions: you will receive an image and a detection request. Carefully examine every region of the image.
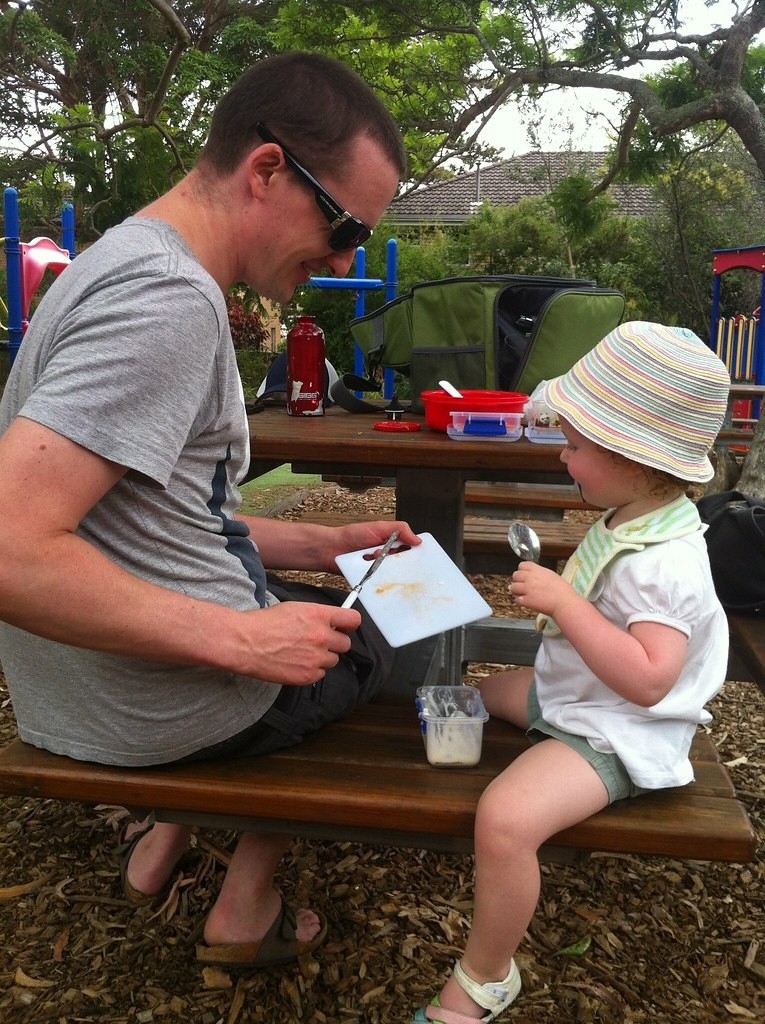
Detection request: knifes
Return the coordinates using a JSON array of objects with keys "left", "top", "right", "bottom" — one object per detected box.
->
[{"left": 331, "top": 531, "right": 400, "bottom": 629}]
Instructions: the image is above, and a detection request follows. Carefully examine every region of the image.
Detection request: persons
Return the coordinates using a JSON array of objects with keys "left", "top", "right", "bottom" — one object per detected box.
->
[
  {"left": 0, "top": 53, "right": 424, "bottom": 966},
  {"left": 404, "top": 321, "right": 732, "bottom": 1024}
]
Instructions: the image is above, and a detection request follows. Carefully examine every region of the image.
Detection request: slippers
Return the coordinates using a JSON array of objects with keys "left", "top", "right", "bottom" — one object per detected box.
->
[
  {"left": 196, "top": 909, "right": 326, "bottom": 964},
  {"left": 112, "top": 818, "right": 153, "bottom": 904}
]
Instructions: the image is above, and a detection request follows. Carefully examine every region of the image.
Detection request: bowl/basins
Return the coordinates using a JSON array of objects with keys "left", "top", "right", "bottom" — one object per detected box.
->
[
  {"left": 447, "top": 411, "right": 524, "bottom": 443},
  {"left": 419, "top": 390, "right": 530, "bottom": 432},
  {"left": 416, "top": 686, "right": 488, "bottom": 768}
]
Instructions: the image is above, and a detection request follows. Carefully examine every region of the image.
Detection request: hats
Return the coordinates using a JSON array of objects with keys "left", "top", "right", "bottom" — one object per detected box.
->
[
  {"left": 543, "top": 321, "right": 732, "bottom": 483},
  {"left": 254, "top": 347, "right": 340, "bottom": 407}
]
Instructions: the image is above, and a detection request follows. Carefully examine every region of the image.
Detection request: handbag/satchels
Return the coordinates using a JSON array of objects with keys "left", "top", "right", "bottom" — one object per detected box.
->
[
  {"left": 695, "top": 489, "right": 765, "bottom": 615},
  {"left": 329, "top": 275, "right": 626, "bottom": 412}
]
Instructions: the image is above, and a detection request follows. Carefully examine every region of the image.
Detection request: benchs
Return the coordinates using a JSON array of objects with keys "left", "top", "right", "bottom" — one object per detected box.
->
[
  {"left": 713, "top": 418, "right": 758, "bottom": 445},
  {"left": 727, "top": 612, "right": 765, "bottom": 694},
  {"left": 0, "top": 472, "right": 765, "bottom": 866}
]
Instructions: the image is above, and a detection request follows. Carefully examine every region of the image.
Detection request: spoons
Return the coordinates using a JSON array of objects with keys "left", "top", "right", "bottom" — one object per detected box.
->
[{"left": 507, "top": 522, "right": 540, "bottom": 565}]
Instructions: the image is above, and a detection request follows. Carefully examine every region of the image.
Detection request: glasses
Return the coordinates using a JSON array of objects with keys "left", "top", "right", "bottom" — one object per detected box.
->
[{"left": 257, "top": 122, "right": 374, "bottom": 253}]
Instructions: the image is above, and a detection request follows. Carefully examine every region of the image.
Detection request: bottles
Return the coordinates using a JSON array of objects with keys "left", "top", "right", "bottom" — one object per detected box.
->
[{"left": 286, "top": 316, "right": 325, "bottom": 416}]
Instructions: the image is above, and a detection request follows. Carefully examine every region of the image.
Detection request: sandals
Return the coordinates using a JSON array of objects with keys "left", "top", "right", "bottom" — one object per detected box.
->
[{"left": 410, "top": 956, "right": 523, "bottom": 1024}]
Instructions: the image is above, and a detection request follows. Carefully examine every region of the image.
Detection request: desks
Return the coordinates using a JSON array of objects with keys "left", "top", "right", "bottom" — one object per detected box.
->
[
  {"left": 722, "top": 383, "right": 765, "bottom": 454},
  {"left": 241, "top": 396, "right": 719, "bottom": 670}
]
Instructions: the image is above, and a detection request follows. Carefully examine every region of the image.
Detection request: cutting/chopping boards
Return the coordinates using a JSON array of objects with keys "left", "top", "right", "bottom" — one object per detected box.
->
[{"left": 335, "top": 532, "right": 493, "bottom": 648}]
